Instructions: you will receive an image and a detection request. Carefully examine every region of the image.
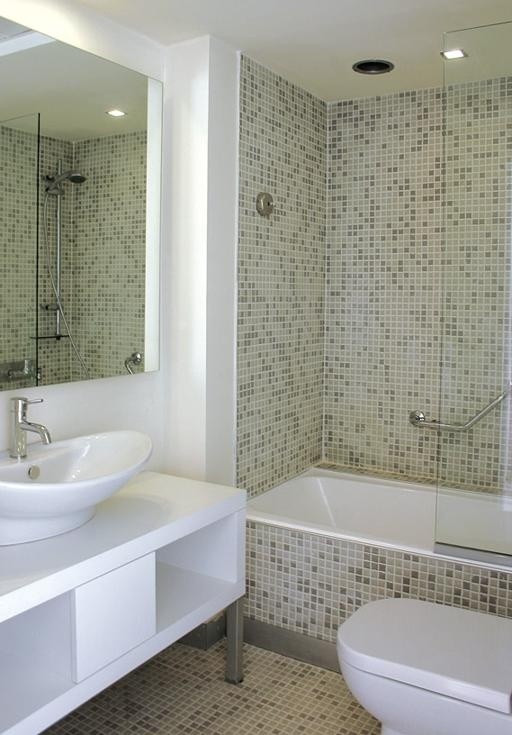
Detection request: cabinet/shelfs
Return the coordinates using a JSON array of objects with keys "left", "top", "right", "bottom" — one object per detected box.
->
[{"left": 1, "top": 472, "right": 248, "bottom": 735}]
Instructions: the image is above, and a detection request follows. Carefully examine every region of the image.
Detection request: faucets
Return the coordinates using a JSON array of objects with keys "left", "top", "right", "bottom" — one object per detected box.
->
[{"left": 8, "top": 397, "right": 51, "bottom": 459}]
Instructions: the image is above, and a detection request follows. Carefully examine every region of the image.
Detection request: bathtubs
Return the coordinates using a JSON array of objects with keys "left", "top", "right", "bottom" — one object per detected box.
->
[{"left": 245, "top": 467, "right": 511, "bottom": 578}]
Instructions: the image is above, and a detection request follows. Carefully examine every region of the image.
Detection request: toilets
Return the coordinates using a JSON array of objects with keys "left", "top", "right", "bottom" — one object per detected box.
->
[{"left": 336, "top": 596, "right": 512, "bottom": 734}]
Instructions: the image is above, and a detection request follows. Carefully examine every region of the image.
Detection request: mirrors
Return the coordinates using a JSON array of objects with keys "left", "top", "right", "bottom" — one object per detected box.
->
[{"left": 0, "top": 17, "right": 166, "bottom": 393}]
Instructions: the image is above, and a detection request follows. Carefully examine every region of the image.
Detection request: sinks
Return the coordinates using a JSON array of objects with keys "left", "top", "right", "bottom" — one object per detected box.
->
[{"left": 0, "top": 430, "right": 153, "bottom": 547}]
